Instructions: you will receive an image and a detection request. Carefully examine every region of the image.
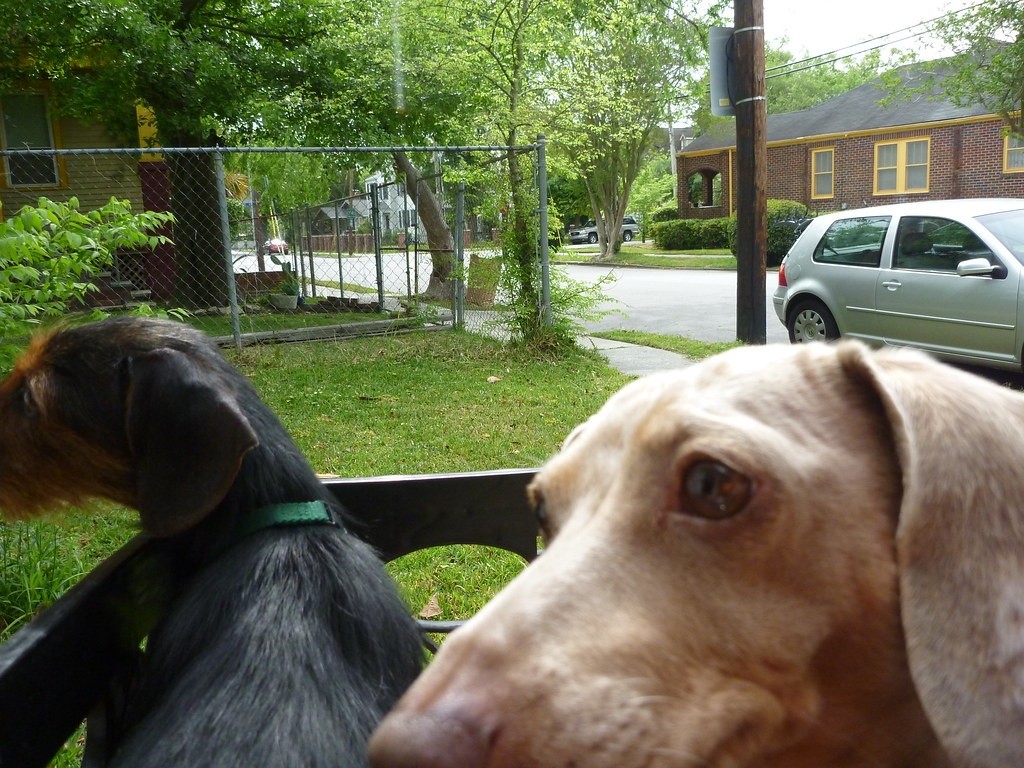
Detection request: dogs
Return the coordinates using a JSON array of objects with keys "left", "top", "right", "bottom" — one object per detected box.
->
[{"left": 0, "top": 315, "right": 1023, "bottom": 768}]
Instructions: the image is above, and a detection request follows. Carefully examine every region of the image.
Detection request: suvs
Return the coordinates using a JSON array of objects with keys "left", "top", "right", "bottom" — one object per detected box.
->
[{"left": 568, "top": 214, "right": 640, "bottom": 244}]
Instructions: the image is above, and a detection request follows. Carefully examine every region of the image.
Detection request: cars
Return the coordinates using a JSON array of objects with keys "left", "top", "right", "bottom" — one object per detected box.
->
[
  {"left": 772, "top": 197, "right": 1024, "bottom": 375},
  {"left": 267, "top": 239, "right": 290, "bottom": 255}
]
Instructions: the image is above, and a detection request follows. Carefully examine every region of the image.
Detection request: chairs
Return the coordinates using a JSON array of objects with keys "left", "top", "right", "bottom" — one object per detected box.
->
[
  {"left": 898, "top": 232, "right": 950, "bottom": 270},
  {"left": 959, "top": 235, "right": 995, "bottom": 264}
]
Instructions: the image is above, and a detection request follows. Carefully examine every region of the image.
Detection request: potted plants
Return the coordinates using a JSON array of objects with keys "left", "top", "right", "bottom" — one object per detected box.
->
[
  {"left": 267, "top": 279, "right": 299, "bottom": 310},
  {"left": 692, "top": 189, "right": 702, "bottom": 207}
]
